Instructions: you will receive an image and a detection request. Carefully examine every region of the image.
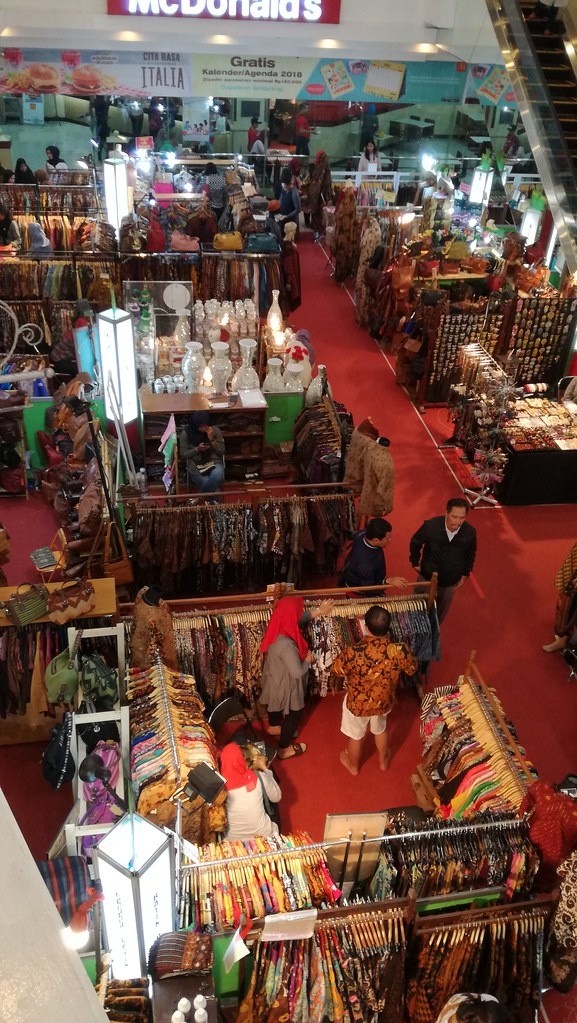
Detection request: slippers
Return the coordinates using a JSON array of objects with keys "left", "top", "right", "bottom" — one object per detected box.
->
[{"left": 276, "top": 742, "right": 306, "bottom": 760}]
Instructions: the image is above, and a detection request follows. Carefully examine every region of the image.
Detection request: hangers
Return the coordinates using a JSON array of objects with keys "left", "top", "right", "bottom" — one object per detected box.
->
[
  {"left": 0, "top": 170, "right": 404, "bottom": 372},
  {"left": 0, "top": 380, "right": 549, "bottom": 962}
]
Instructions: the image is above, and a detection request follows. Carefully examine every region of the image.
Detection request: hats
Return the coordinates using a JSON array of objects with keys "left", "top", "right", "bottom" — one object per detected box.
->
[
  {"left": 251, "top": 117, "right": 263, "bottom": 124},
  {"left": 506, "top": 124, "right": 517, "bottom": 130}
]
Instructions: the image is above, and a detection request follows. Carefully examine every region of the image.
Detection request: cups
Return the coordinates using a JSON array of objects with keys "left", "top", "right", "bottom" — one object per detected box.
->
[
  {"left": 61, "top": 51, "right": 80, "bottom": 84},
  {"left": 153, "top": 375, "right": 186, "bottom": 394},
  {"left": 4, "top": 47, "right": 23, "bottom": 82}
]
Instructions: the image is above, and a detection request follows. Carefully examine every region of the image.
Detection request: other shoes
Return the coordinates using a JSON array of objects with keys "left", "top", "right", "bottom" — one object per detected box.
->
[
  {"left": 204, "top": 500, "right": 218, "bottom": 506},
  {"left": 186, "top": 498, "right": 196, "bottom": 506}
]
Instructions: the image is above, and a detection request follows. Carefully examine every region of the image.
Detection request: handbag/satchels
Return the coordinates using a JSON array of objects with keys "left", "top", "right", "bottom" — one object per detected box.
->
[
  {"left": 83, "top": 739, "right": 121, "bottom": 804},
  {"left": 3, "top": 582, "right": 51, "bottom": 628},
  {"left": 77, "top": 790, "right": 126, "bottom": 858},
  {"left": 374, "top": 126, "right": 385, "bottom": 138},
  {"left": 76, "top": 483, "right": 105, "bottom": 536},
  {"left": 81, "top": 651, "right": 119, "bottom": 710},
  {"left": 82, "top": 521, "right": 119, "bottom": 579},
  {"left": 45, "top": 575, "right": 96, "bottom": 626},
  {"left": 38, "top": 711, "right": 76, "bottom": 791},
  {"left": 335, "top": 530, "right": 365, "bottom": 574},
  {"left": 44, "top": 628, "right": 84, "bottom": 704},
  {"left": 103, "top": 521, "right": 133, "bottom": 585},
  {"left": 254, "top": 770, "right": 275, "bottom": 816}
]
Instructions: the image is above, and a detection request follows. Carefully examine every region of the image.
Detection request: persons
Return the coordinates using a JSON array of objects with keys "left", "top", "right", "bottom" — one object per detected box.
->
[
  {"left": 179, "top": 410, "right": 226, "bottom": 506},
  {"left": 503, "top": 124, "right": 520, "bottom": 159},
  {"left": 409, "top": 497, "right": 477, "bottom": 626},
  {"left": 340, "top": 517, "right": 408, "bottom": 600},
  {"left": 259, "top": 595, "right": 338, "bottom": 760},
  {"left": 0, "top": 145, "right": 68, "bottom": 186},
  {"left": 247, "top": 116, "right": 265, "bottom": 165},
  {"left": 358, "top": 138, "right": 383, "bottom": 183},
  {"left": 329, "top": 605, "right": 419, "bottom": 776},
  {"left": 435, "top": 992, "right": 511, "bottom": 1023},
  {"left": 278, "top": 158, "right": 315, "bottom": 244},
  {"left": 183, "top": 119, "right": 209, "bottom": 135},
  {"left": 0, "top": 203, "right": 54, "bottom": 261},
  {"left": 219, "top": 742, "right": 282, "bottom": 842},
  {"left": 90, "top": 95, "right": 177, "bottom": 161},
  {"left": 294, "top": 101, "right": 318, "bottom": 156},
  {"left": 359, "top": 103, "right": 380, "bottom": 156},
  {"left": 541, "top": 569, "right": 577, "bottom": 653},
  {"left": 201, "top": 162, "right": 229, "bottom": 223}
]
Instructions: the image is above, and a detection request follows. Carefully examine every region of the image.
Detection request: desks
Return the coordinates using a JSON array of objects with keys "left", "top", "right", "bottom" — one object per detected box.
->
[{"left": 472, "top": 396, "right": 577, "bottom": 506}]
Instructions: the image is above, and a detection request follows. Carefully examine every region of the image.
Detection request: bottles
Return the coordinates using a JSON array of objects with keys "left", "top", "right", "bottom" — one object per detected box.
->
[
  {"left": 194, "top": 1008, "right": 208, "bottom": 1023},
  {"left": 193, "top": 994, "right": 207, "bottom": 1011},
  {"left": 193, "top": 298, "right": 256, "bottom": 319},
  {"left": 195, "top": 320, "right": 257, "bottom": 337},
  {"left": 171, "top": 1011, "right": 185, "bottom": 1023},
  {"left": 137, "top": 468, "right": 148, "bottom": 498},
  {"left": 177, "top": 998, "right": 191, "bottom": 1021}
]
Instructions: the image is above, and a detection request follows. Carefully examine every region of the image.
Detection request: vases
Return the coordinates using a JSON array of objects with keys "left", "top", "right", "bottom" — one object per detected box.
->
[
  {"left": 181, "top": 341, "right": 206, "bottom": 394},
  {"left": 285, "top": 364, "right": 304, "bottom": 392},
  {"left": 264, "top": 358, "right": 283, "bottom": 392},
  {"left": 209, "top": 343, "right": 231, "bottom": 395},
  {"left": 232, "top": 338, "right": 259, "bottom": 393},
  {"left": 306, "top": 365, "right": 332, "bottom": 407}
]
